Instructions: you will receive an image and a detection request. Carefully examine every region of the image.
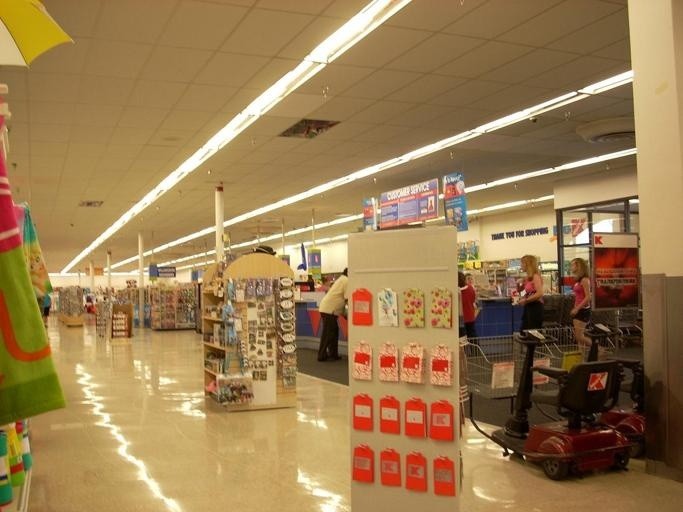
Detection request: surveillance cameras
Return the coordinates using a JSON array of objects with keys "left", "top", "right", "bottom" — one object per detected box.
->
[{"left": 530, "top": 118, "right": 538, "bottom": 123}]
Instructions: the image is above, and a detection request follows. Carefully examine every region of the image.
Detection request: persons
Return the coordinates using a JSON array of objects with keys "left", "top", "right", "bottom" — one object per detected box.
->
[
  {"left": 570, "top": 258, "right": 606, "bottom": 356},
  {"left": 43, "top": 294, "right": 51, "bottom": 328},
  {"left": 511, "top": 255, "right": 545, "bottom": 336},
  {"left": 458, "top": 271, "right": 480, "bottom": 354},
  {"left": 308, "top": 273, "right": 315, "bottom": 292},
  {"left": 84, "top": 295, "right": 96, "bottom": 320},
  {"left": 318, "top": 267, "right": 348, "bottom": 362},
  {"left": 429, "top": 200, "right": 433, "bottom": 210}
]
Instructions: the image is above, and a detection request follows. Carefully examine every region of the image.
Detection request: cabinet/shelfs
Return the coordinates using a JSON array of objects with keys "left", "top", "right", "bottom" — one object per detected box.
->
[
  {"left": 57, "top": 282, "right": 198, "bottom": 340},
  {"left": 197, "top": 252, "right": 300, "bottom": 409}
]
